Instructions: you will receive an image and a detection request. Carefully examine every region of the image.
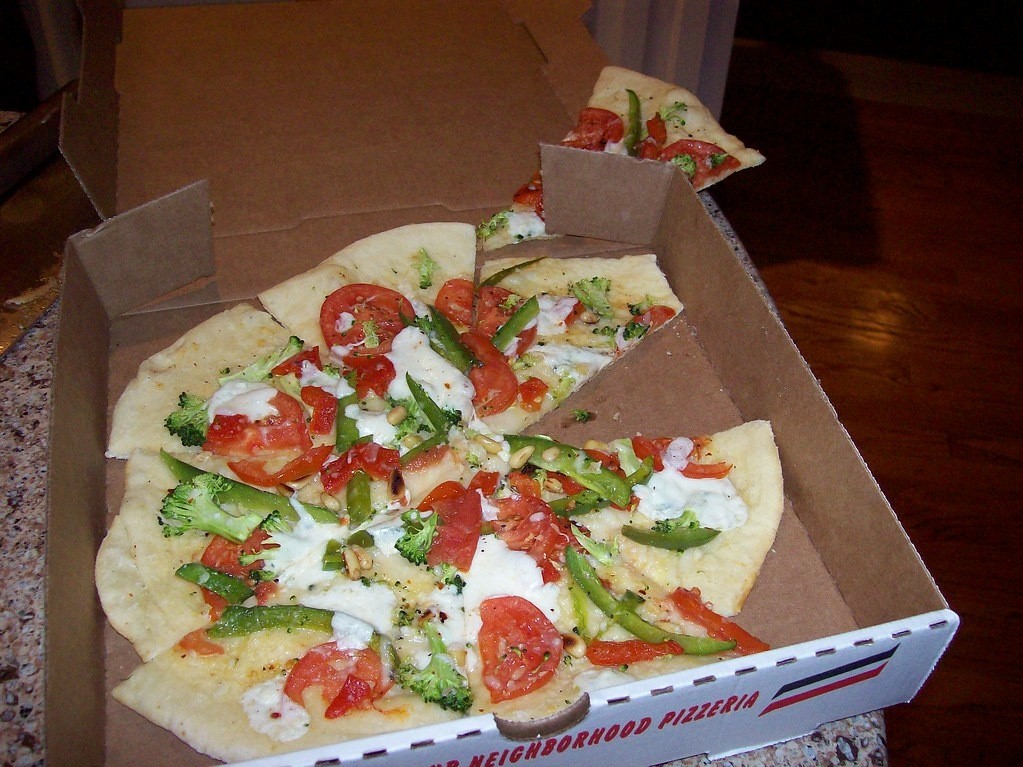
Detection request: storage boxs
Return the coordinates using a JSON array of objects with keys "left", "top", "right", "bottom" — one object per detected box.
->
[{"left": 41, "top": 1, "right": 960, "bottom": 767}]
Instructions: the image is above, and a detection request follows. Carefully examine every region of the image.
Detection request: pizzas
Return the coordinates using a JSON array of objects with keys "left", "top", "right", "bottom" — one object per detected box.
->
[
  {"left": 93, "top": 220, "right": 783, "bottom": 764},
  {"left": 475, "top": 66, "right": 765, "bottom": 250}
]
